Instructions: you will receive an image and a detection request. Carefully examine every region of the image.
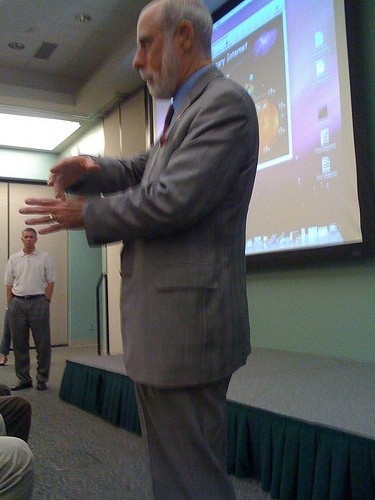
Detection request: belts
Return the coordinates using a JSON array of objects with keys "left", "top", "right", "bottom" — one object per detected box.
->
[{"left": 13, "top": 294, "right": 44, "bottom": 299}]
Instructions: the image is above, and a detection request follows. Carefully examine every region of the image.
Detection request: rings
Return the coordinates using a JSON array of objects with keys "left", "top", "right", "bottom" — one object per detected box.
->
[{"left": 49, "top": 214, "right": 55, "bottom": 221}]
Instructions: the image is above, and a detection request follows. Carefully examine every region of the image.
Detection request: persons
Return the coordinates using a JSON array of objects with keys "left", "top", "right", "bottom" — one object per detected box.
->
[
  {"left": 0, "top": 383, "right": 36, "bottom": 500},
  {"left": 0, "top": 312, "right": 11, "bottom": 365},
  {"left": 5, "top": 227, "right": 55, "bottom": 389},
  {"left": 19, "top": 0, "right": 260, "bottom": 500}
]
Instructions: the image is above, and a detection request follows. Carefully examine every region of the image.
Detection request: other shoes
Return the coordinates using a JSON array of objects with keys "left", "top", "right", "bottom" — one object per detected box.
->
[
  {"left": 36, "top": 382, "right": 46, "bottom": 390},
  {"left": 10, "top": 382, "right": 32, "bottom": 391},
  {"left": 0, "top": 358, "right": 7, "bottom": 365}
]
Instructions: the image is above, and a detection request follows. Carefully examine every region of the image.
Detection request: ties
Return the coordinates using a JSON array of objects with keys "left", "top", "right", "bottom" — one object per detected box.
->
[{"left": 159, "top": 105, "right": 175, "bottom": 147}]
